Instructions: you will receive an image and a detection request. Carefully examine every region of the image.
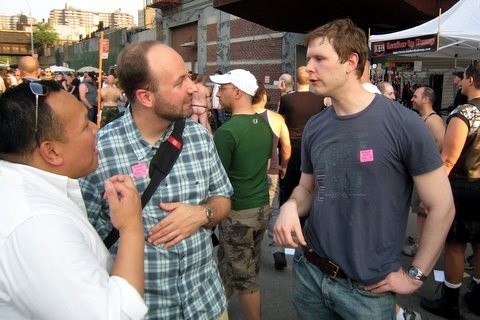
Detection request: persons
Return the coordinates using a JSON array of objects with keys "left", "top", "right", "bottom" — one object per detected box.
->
[
  {"left": 361, "top": 59, "right": 383, "bottom": 94},
  {"left": 446, "top": 71, "right": 469, "bottom": 114},
  {"left": 275, "top": 65, "right": 325, "bottom": 210},
  {"left": 274, "top": 20, "right": 456, "bottom": 320},
  {"left": 418, "top": 64, "right": 480, "bottom": 320},
  {"left": 78, "top": 39, "right": 235, "bottom": 320},
  {"left": 185, "top": 69, "right": 223, "bottom": 136},
  {"left": 401, "top": 87, "right": 446, "bottom": 258},
  {"left": 0, "top": 80, "right": 150, "bottom": 320},
  {"left": 251, "top": 81, "right": 291, "bottom": 271},
  {"left": 377, "top": 82, "right": 396, "bottom": 101},
  {"left": 207, "top": 68, "right": 273, "bottom": 320},
  {"left": 0, "top": 56, "right": 130, "bottom": 129}
]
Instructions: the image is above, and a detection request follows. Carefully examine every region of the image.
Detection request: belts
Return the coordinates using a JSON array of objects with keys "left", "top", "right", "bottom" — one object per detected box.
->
[{"left": 301, "top": 243, "right": 358, "bottom": 283}]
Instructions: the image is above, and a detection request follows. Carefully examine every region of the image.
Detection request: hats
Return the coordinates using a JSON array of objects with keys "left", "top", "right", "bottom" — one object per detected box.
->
[
  {"left": 452, "top": 71, "right": 464, "bottom": 80},
  {"left": 209, "top": 69, "right": 258, "bottom": 96},
  {"left": 89, "top": 71, "right": 97, "bottom": 80}
]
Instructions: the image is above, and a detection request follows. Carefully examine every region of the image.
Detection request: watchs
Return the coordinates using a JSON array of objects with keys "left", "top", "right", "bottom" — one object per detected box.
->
[
  {"left": 203, "top": 205, "right": 214, "bottom": 225},
  {"left": 408, "top": 265, "right": 428, "bottom": 283}
]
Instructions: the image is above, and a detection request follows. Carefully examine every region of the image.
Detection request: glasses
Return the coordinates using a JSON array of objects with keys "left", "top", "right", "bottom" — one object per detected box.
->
[
  {"left": 471, "top": 58, "right": 480, "bottom": 76},
  {"left": 217, "top": 87, "right": 237, "bottom": 91},
  {"left": 22, "top": 77, "right": 48, "bottom": 147}
]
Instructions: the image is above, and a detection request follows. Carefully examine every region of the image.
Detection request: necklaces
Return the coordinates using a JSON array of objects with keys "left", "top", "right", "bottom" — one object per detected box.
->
[{"left": 287, "top": 90, "right": 294, "bottom": 94}]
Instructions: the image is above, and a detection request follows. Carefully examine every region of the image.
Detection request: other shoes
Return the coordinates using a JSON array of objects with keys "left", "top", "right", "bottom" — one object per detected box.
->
[
  {"left": 274, "top": 252, "right": 287, "bottom": 270},
  {"left": 400, "top": 302, "right": 422, "bottom": 320},
  {"left": 401, "top": 239, "right": 420, "bottom": 257},
  {"left": 462, "top": 254, "right": 476, "bottom": 270}
]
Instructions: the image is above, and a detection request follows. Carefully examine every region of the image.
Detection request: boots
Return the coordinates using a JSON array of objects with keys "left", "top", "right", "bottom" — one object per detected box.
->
[
  {"left": 460, "top": 274, "right": 480, "bottom": 317},
  {"left": 420, "top": 281, "right": 462, "bottom": 320}
]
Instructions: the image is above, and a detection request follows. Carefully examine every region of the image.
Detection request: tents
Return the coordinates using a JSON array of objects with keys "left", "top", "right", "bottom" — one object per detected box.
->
[{"left": 368, "top": 0, "right": 480, "bottom": 76}]
